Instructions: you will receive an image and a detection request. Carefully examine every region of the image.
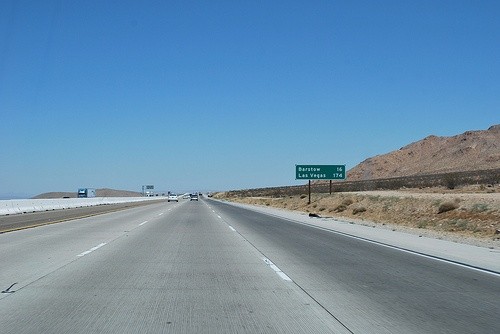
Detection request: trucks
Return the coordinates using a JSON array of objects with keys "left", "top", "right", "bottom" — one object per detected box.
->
[{"left": 77, "top": 188, "right": 96, "bottom": 198}]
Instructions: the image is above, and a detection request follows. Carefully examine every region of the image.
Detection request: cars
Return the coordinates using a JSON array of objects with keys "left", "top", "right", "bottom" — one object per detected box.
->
[
  {"left": 191, "top": 194, "right": 198, "bottom": 201},
  {"left": 183, "top": 192, "right": 212, "bottom": 199},
  {"left": 168, "top": 193, "right": 178, "bottom": 202}
]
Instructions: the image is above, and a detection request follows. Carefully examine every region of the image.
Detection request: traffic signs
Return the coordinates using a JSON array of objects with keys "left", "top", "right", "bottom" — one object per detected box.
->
[{"left": 294, "top": 164, "right": 346, "bottom": 180}]
